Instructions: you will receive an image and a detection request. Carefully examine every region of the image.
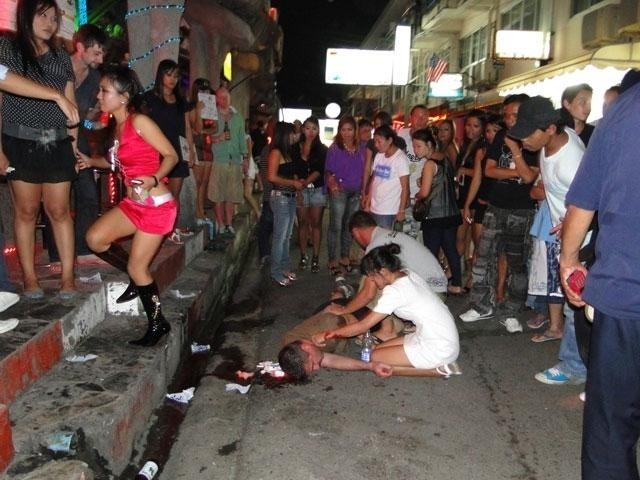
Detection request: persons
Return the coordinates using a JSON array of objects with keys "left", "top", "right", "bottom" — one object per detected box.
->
[
  {"left": 76, "top": 64, "right": 179, "bottom": 346},
  {"left": 187, "top": 66, "right": 639, "bottom": 387},
  {"left": 560, "top": 80, "right": 640, "bottom": 479},
  {"left": 138, "top": 58, "right": 194, "bottom": 244},
  {"left": 0, "top": 63, "right": 81, "bottom": 332},
  {"left": 103, "top": 38, "right": 131, "bottom": 67},
  {"left": 0, "top": 0, "right": 78, "bottom": 305},
  {"left": 71, "top": 23, "right": 105, "bottom": 249}
]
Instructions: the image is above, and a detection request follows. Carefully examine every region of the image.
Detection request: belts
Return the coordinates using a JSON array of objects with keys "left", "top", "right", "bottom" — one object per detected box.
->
[{"left": 282, "top": 193, "right": 296, "bottom": 197}]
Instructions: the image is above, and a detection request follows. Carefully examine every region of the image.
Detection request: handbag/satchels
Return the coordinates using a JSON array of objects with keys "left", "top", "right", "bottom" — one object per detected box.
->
[{"left": 412, "top": 200, "right": 431, "bottom": 221}]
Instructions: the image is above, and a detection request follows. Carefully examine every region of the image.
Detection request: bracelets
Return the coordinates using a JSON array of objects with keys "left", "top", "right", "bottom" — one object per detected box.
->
[
  {"left": 65, "top": 122, "right": 81, "bottom": 129},
  {"left": 244, "top": 155, "right": 247, "bottom": 159},
  {"left": 151, "top": 175, "right": 158, "bottom": 185}
]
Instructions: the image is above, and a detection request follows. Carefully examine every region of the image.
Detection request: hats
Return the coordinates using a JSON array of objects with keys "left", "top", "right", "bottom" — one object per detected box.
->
[
  {"left": 509, "top": 97, "right": 555, "bottom": 138},
  {"left": 196, "top": 78, "right": 214, "bottom": 93}
]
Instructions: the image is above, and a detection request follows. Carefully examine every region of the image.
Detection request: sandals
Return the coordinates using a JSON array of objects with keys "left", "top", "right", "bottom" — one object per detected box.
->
[{"left": 436, "top": 363, "right": 462, "bottom": 376}]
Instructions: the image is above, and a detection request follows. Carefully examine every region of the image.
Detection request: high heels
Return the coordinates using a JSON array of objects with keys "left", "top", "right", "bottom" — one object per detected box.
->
[
  {"left": 168, "top": 224, "right": 235, "bottom": 245},
  {"left": 271, "top": 255, "right": 352, "bottom": 286}
]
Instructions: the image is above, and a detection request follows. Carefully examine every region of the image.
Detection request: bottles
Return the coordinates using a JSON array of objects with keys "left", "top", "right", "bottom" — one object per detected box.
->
[{"left": 361, "top": 328, "right": 374, "bottom": 363}]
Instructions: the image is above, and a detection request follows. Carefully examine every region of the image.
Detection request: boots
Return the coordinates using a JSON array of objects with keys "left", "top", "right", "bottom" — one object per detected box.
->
[
  {"left": 129, "top": 279, "right": 171, "bottom": 347},
  {"left": 95, "top": 242, "right": 139, "bottom": 303}
]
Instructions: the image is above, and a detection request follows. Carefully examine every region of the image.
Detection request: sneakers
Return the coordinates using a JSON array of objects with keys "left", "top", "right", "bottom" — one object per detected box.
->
[
  {"left": 535, "top": 368, "right": 569, "bottom": 384},
  {"left": 447, "top": 287, "right": 562, "bottom": 342},
  {"left": 0, "top": 318, "right": 19, "bottom": 334}
]
